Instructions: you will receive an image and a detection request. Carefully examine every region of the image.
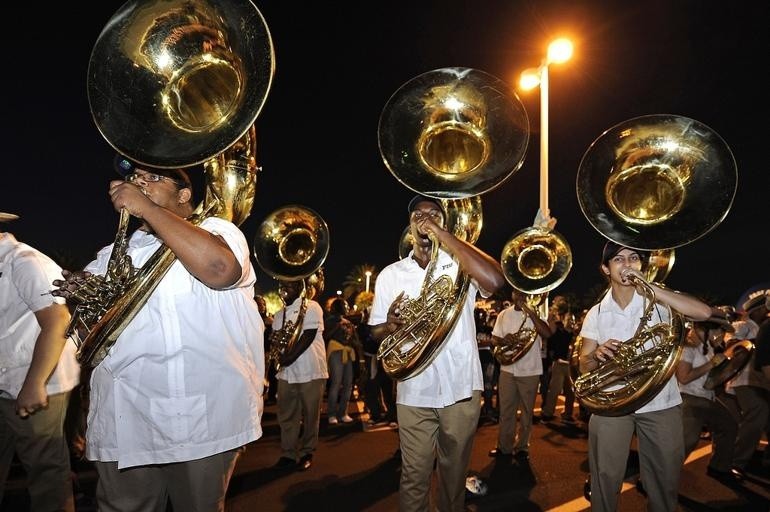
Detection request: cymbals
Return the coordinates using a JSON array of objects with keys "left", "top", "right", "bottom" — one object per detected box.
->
[{"left": 704, "top": 340, "right": 755, "bottom": 391}]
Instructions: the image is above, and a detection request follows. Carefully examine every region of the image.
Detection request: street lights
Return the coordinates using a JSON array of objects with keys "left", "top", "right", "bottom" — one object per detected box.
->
[
  {"left": 364, "top": 267, "right": 373, "bottom": 294},
  {"left": 518, "top": 37, "right": 574, "bottom": 381}
]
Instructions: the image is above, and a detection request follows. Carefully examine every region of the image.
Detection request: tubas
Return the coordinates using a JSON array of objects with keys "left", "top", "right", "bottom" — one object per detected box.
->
[
  {"left": 46, "top": 0, "right": 275, "bottom": 367},
  {"left": 255, "top": 207, "right": 331, "bottom": 379},
  {"left": 377, "top": 66, "right": 530, "bottom": 381},
  {"left": 493, "top": 228, "right": 572, "bottom": 364},
  {"left": 568, "top": 114, "right": 740, "bottom": 416}
]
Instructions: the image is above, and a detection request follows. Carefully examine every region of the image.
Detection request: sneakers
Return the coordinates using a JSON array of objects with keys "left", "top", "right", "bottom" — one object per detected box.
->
[
  {"left": 329, "top": 416, "right": 338, "bottom": 424},
  {"left": 341, "top": 416, "right": 352, "bottom": 423}
]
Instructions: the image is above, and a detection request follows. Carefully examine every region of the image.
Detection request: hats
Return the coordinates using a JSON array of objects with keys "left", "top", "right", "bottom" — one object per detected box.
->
[
  {"left": 603, "top": 241, "right": 643, "bottom": 263},
  {"left": 408, "top": 195, "right": 447, "bottom": 225}
]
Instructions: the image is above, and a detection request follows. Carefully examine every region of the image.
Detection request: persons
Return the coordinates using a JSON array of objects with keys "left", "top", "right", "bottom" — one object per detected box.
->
[
  {"left": 254, "top": 279, "right": 770, "bottom": 478},
  {"left": 371, "top": 193, "right": 502, "bottom": 508},
  {"left": 0, "top": 218, "right": 84, "bottom": 510},
  {"left": 579, "top": 241, "right": 712, "bottom": 511},
  {"left": 62, "top": 163, "right": 269, "bottom": 509}
]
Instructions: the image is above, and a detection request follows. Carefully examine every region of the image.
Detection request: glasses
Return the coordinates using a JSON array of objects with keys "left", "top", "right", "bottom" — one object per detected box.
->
[{"left": 125, "top": 174, "right": 184, "bottom": 183}]
